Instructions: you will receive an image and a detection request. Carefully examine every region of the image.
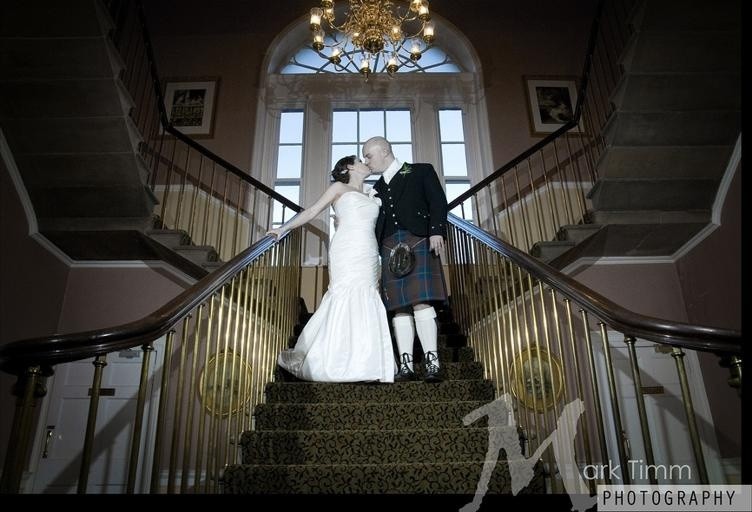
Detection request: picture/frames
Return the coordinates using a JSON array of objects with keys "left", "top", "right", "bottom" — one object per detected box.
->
[
  {"left": 519, "top": 72, "right": 591, "bottom": 139},
  {"left": 151, "top": 75, "right": 221, "bottom": 141}
]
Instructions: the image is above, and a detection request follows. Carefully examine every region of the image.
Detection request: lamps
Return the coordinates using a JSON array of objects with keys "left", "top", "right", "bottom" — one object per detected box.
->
[{"left": 302, "top": 0, "right": 439, "bottom": 84}]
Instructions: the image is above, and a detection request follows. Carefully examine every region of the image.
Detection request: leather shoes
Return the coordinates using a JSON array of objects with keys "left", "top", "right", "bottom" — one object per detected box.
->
[{"left": 394, "top": 366, "right": 418, "bottom": 381}]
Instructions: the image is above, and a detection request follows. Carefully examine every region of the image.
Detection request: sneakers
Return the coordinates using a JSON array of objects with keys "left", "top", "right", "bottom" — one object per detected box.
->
[{"left": 423, "top": 364, "right": 444, "bottom": 382}]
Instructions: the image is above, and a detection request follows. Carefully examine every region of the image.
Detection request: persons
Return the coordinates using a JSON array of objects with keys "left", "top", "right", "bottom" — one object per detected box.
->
[
  {"left": 267, "top": 154, "right": 398, "bottom": 384},
  {"left": 362, "top": 136, "right": 448, "bottom": 383}
]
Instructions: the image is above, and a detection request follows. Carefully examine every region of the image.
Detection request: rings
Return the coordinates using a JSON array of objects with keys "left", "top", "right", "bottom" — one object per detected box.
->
[{"left": 438, "top": 243, "right": 440, "bottom": 247}]
